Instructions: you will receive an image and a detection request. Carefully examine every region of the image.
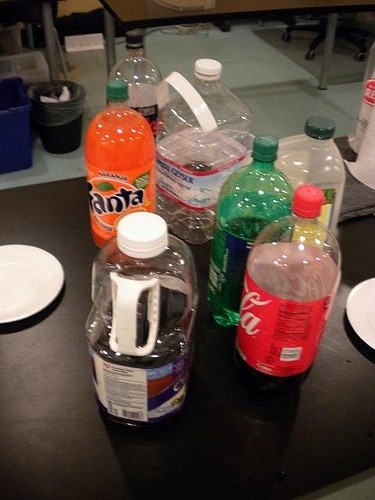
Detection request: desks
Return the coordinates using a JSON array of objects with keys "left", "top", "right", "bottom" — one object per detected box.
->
[
  {"left": 0, "top": 175, "right": 375, "bottom": 500},
  {"left": 39, "top": 0, "right": 375, "bottom": 89}
]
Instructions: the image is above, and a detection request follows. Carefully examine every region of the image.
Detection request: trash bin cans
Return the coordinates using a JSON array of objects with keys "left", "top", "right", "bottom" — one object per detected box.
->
[{"left": 28, "top": 80, "right": 84, "bottom": 154}]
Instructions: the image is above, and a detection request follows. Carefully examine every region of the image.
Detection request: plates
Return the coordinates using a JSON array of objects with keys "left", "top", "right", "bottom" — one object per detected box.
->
[
  {"left": 0, "top": 242, "right": 65, "bottom": 325},
  {"left": 345, "top": 277, "right": 375, "bottom": 350}
]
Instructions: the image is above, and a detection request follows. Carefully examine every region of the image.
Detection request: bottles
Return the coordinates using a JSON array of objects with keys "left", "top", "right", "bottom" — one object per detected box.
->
[
  {"left": 234, "top": 184, "right": 342, "bottom": 378},
  {"left": 84, "top": 78, "right": 157, "bottom": 248},
  {"left": 109, "top": 28, "right": 161, "bottom": 144},
  {"left": 154, "top": 58, "right": 254, "bottom": 247},
  {"left": 274, "top": 114, "right": 346, "bottom": 254},
  {"left": 206, "top": 135, "right": 294, "bottom": 328},
  {"left": 85, "top": 212, "right": 198, "bottom": 428}
]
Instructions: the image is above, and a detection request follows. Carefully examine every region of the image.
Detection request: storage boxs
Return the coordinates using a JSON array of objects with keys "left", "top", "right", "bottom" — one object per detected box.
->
[{"left": 0, "top": 77, "right": 33, "bottom": 174}]
